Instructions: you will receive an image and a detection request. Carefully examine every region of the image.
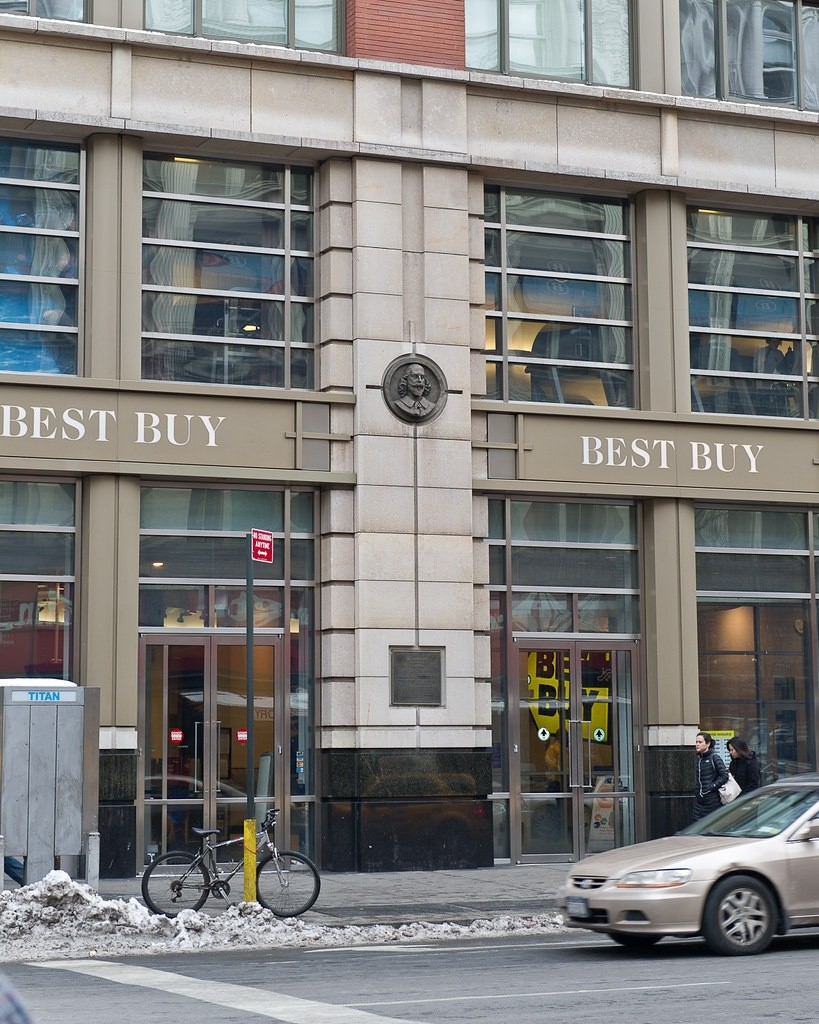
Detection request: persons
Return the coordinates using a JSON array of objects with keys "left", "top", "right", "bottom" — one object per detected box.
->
[
  {"left": 394, "top": 363, "right": 436, "bottom": 418},
  {"left": 545, "top": 729, "right": 568, "bottom": 840},
  {"left": 692, "top": 732, "right": 729, "bottom": 821},
  {"left": 727, "top": 736, "right": 760, "bottom": 824},
  {"left": 752, "top": 338, "right": 786, "bottom": 390}
]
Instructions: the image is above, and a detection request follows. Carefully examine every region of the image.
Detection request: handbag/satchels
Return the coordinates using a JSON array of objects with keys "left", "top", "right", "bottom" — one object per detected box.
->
[{"left": 717, "top": 771, "right": 742, "bottom": 805}]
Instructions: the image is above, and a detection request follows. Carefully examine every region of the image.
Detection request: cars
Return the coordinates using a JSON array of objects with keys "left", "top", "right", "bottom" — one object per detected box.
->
[{"left": 562, "top": 773, "right": 819, "bottom": 956}]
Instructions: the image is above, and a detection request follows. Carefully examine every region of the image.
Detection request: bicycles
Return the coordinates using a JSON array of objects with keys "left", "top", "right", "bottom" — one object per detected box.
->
[{"left": 141, "top": 809, "right": 321, "bottom": 919}]
